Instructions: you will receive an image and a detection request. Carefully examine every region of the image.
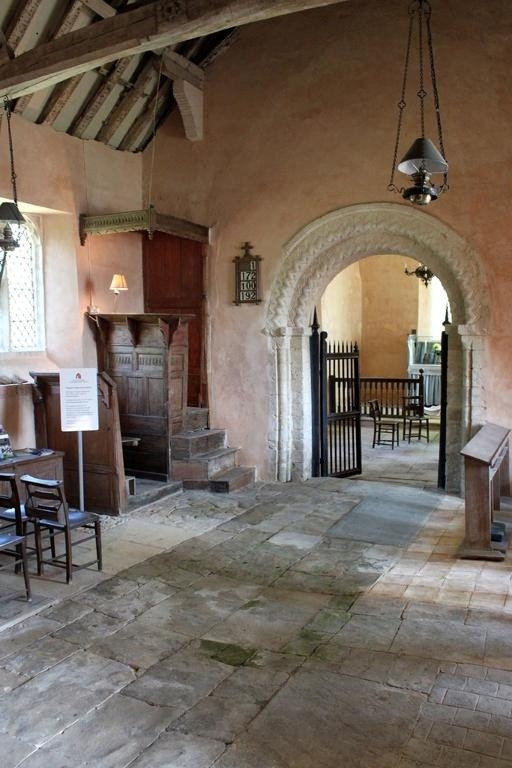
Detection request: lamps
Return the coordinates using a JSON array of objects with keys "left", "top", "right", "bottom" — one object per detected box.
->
[
  {"left": 108, "top": 273, "right": 128, "bottom": 312},
  {"left": 1, "top": 95, "right": 26, "bottom": 250},
  {"left": 404, "top": 257, "right": 435, "bottom": 288},
  {"left": 386, "top": 0, "right": 450, "bottom": 207}
]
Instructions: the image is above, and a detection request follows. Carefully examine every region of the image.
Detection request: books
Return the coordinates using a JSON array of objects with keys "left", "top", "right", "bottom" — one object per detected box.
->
[{"left": 0, "top": 424, "right": 15, "bottom": 461}]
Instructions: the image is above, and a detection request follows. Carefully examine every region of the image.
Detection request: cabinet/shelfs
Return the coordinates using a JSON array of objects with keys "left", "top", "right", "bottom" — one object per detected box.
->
[{"left": 0, "top": 458, "right": 66, "bottom": 503}]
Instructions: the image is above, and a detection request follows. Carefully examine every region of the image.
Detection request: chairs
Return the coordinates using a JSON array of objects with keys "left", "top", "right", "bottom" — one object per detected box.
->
[
  {"left": 88, "top": 230, "right": 203, "bottom": 407},
  {"left": 367, "top": 395, "right": 433, "bottom": 450},
  {"left": 0, "top": 471, "right": 103, "bottom": 603}
]
